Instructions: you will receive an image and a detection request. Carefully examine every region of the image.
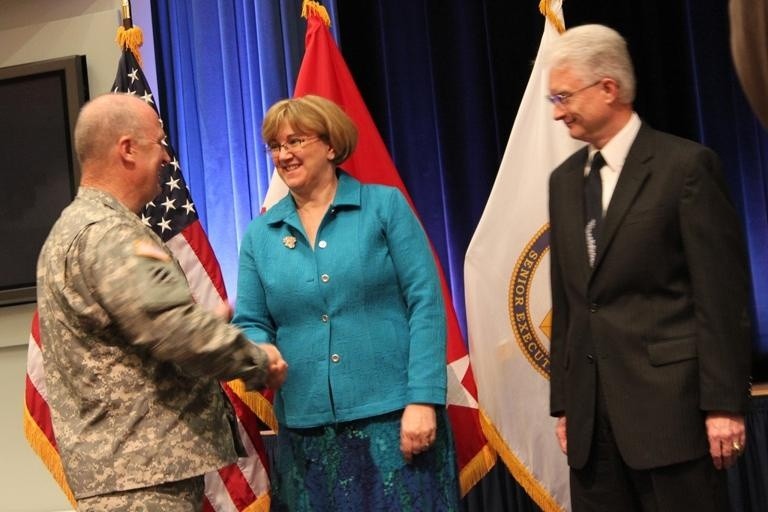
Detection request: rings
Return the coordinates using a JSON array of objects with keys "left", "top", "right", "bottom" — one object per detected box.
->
[
  {"left": 731, "top": 439, "right": 745, "bottom": 456},
  {"left": 420, "top": 439, "right": 430, "bottom": 446}
]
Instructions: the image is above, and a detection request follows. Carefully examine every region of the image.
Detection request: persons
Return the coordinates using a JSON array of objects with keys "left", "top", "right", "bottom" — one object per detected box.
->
[
  {"left": 543, "top": 22, "right": 759, "bottom": 511},
  {"left": 34, "top": 89, "right": 290, "bottom": 512},
  {"left": 221, "top": 93, "right": 466, "bottom": 512}
]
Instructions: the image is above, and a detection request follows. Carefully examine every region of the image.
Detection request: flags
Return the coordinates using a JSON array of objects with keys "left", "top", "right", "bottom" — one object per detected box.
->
[
  {"left": 17, "top": 21, "right": 282, "bottom": 512},
  {"left": 462, "top": 0, "right": 591, "bottom": 512},
  {"left": 221, "top": 2, "right": 502, "bottom": 511}
]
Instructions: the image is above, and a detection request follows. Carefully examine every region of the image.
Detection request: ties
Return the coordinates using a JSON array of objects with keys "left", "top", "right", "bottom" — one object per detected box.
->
[{"left": 583, "top": 151, "right": 608, "bottom": 269}]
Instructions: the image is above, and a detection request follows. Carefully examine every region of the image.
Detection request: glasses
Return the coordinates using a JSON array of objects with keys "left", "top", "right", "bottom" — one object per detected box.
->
[
  {"left": 263, "top": 134, "right": 322, "bottom": 159},
  {"left": 546, "top": 80, "right": 601, "bottom": 103}
]
